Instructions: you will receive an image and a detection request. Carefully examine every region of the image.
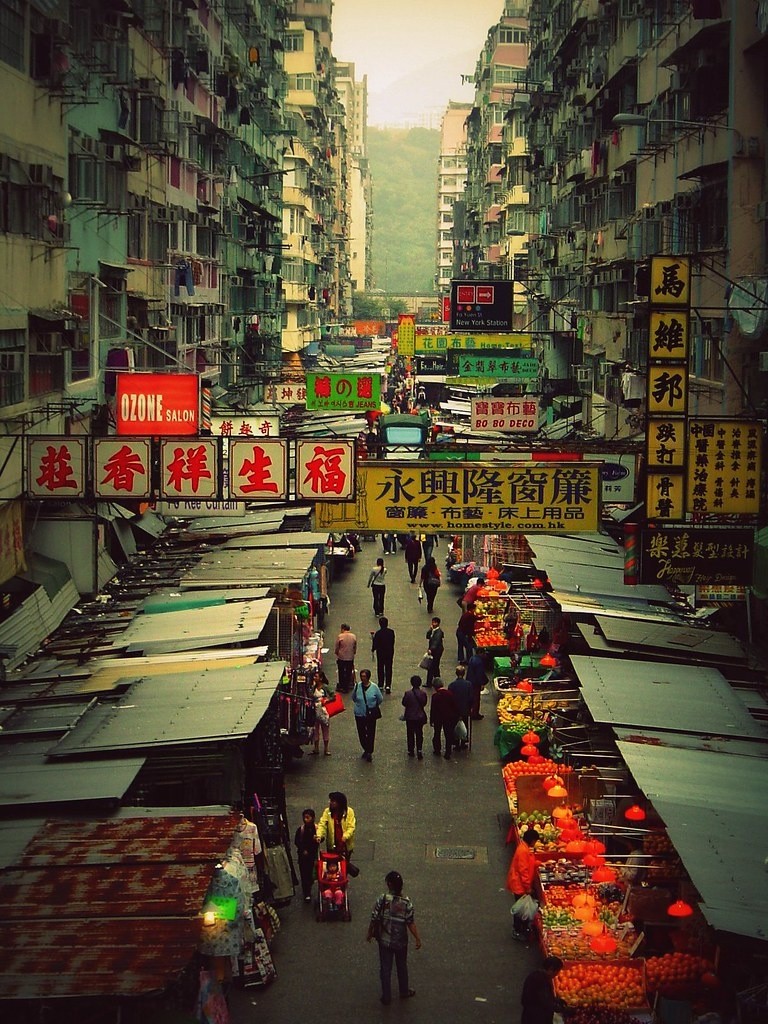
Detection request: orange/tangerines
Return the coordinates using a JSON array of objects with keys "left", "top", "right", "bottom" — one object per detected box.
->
[
  {"left": 507, "top": 722, "right": 540, "bottom": 733},
  {"left": 545, "top": 911, "right": 618, "bottom": 926}
]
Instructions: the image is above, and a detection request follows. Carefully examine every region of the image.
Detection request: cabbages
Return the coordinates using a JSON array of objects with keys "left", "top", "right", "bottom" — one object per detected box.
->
[{"left": 517, "top": 810, "right": 550, "bottom": 822}]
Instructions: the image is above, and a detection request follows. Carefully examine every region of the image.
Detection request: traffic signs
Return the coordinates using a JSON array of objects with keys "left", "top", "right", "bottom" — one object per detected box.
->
[{"left": 450, "top": 280, "right": 514, "bottom": 334}]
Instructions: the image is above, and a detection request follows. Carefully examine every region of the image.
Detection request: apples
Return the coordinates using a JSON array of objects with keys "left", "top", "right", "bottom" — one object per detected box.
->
[
  {"left": 646, "top": 952, "right": 698, "bottom": 982},
  {"left": 504, "top": 758, "right": 573, "bottom": 779},
  {"left": 555, "top": 963, "right": 644, "bottom": 1009}
]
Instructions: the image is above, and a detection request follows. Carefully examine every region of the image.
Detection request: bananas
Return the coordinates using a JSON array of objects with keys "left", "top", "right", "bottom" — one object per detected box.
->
[{"left": 498, "top": 693, "right": 568, "bottom": 723}]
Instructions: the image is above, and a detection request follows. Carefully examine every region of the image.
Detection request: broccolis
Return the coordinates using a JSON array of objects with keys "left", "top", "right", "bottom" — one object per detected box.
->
[{"left": 517, "top": 823, "right": 553, "bottom": 837}]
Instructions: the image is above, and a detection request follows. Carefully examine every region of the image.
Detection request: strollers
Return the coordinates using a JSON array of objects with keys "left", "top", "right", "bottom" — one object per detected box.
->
[{"left": 314, "top": 839, "right": 353, "bottom": 923}]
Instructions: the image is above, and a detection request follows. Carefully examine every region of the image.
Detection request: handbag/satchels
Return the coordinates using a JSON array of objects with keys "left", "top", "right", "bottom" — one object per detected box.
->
[
  {"left": 321, "top": 692, "right": 346, "bottom": 718},
  {"left": 428, "top": 577, "right": 440, "bottom": 587},
  {"left": 424, "top": 711, "right": 427, "bottom": 724},
  {"left": 417, "top": 652, "right": 434, "bottom": 669},
  {"left": 418, "top": 587, "right": 423, "bottom": 603},
  {"left": 366, "top": 706, "right": 382, "bottom": 720},
  {"left": 455, "top": 720, "right": 467, "bottom": 740},
  {"left": 371, "top": 894, "right": 386, "bottom": 938}
]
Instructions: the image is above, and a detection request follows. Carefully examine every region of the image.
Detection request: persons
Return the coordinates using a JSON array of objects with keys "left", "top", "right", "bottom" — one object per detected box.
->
[
  {"left": 460, "top": 578, "right": 485, "bottom": 614},
  {"left": 307, "top": 671, "right": 334, "bottom": 756},
  {"left": 456, "top": 603, "right": 477, "bottom": 665},
  {"left": 405, "top": 535, "right": 422, "bottom": 583},
  {"left": 506, "top": 829, "right": 546, "bottom": 942},
  {"left": 335, "top": 623, "right": 357, "bottom": 692},
  {"left": 294, "top": 809, "right": 325, "bottom": 903},
  {"left": 322, "top": 859, "right": 344, "bottom": 911},
  {"left": 402, "top": 675, "right": 427, "bottom": 761},
  {"left": 371, "top": 617, "right": 395, "bottom": 695},
  {"left": 422, "top": 534, "right": 439, "bottom": 565},
  {"left": 381, "top": 533, "right": 411, "bottom": 554},
  {"left": 367, "top": 871, "right": 421, "bottom": 1005},
  {"left": 429, "top": 677, "right": 461, "bottom": 760},
  {"left": 618, "top": 835, "right": 647, "bottom": 885},
  {"left": 448, "top": 665, "right": 475, "bottom": 752},
  {"left": 367, "top": 558, "right": 388, "bottom": 617},
  {"left": 521, "top": 956, "right": 568, "bottom": 1024},
  {"left": 466, "top": 647, "right": 489, "bottom": 720},
  {"left": 352, "top": 669, "right": 383, "bottom": 762},
  {"left": 422, "top": 617, "right": 445, "bottom": 688},
  {"left": 315, "top": 792, "right": 356, "bottom": 878},
  {"left": 420, "top": 557, "right": 441, "bottom": 614}
]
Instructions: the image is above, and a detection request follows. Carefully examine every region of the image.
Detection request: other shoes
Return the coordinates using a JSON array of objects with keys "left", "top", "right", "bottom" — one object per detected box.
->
[
  {"left": 381, "top": 995, "right": 391, "bottom": 1004},
  {"left": 379, "top": 613, "right": 383, "bottom": 615},
  {"left": 386, "top": 687, "right": 390, "bottom": 694},
  {"left": 472, "top": 714, "right": 484, "bottom": 720},
  {"left": 391, "top": 551, "right": 395, "bottom": 554},
  {"left": 361, "top": 751, "right": 372, "bottom": 762},
  {"left": 444, "top": 754, "right": 450, "bottom": 760},
  {"left": 401, "top": 987, "right": 415, "bottom": 998},
  {"left": 379, "top": 687, "right": 383, "bottom": 690},
  {"left": 385, "top": 552, "right": 389, "bottom": 554},
  {"left": 375, "top": 614, "right": 379, "bottom": 618},
  {"left": 327, "top": 905, "right": 333, "bottom": 911},
  {"left": 334, "top": 905, "right": 339, "bottom": 910},
  {"left": 433, "top": 751, "right": 441, "bottom": 756},
  {"left": 408, "top": 752, "right": 414, "bottom": 757},
  {"left": 310, "top": 750, "right": 319, "bottom": 755},
  {"left": 304, "top": 895, "right": 311, "bottom": 902},
  {"left": 417, "top": 752, "right": 423, "bottom": 760},
  {"left": 422, "top": 684, "right": 432, "bottom": 688},
  {"left": 324, "top": 750, "right": 331, "bottom": 755}
]
